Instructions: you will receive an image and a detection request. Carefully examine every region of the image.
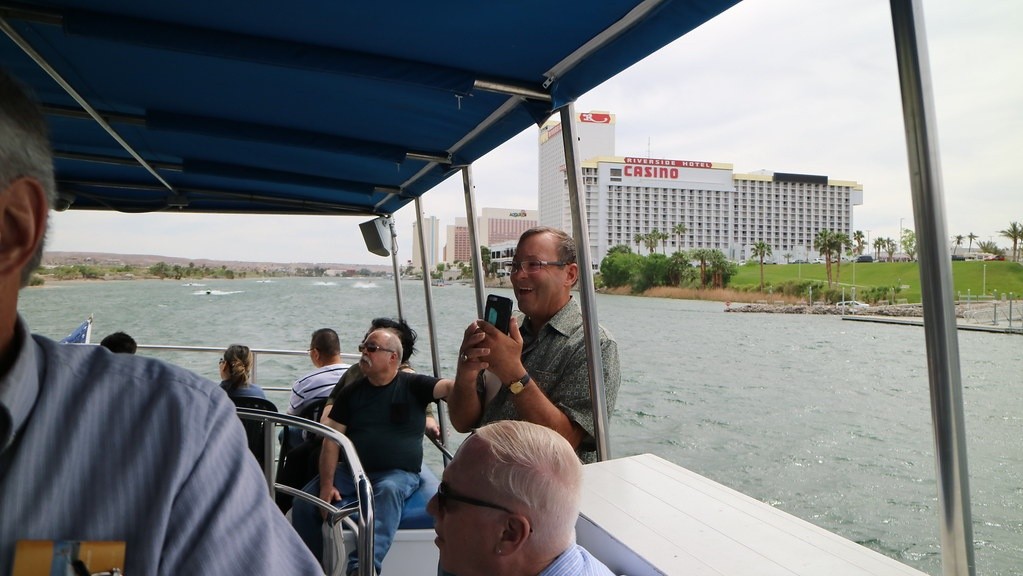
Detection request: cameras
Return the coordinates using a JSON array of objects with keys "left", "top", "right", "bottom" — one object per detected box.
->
[{"left": 484, "top": 293, "right": 513, "bottom": 336}]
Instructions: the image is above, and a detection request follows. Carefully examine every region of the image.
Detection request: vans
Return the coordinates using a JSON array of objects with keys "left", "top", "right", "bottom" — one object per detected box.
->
[{"left": 854, "top": 255, "right": 873, "bottom": 263}]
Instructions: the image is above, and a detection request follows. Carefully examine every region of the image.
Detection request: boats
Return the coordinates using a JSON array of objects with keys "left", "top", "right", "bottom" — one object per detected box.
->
[{"left": 207, "top": 290, "right": 210, "bottom": 293}]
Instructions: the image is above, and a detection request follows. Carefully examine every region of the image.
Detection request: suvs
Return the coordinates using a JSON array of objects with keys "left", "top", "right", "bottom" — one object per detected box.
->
[{"left": 836, "top": 301, "right": 869, "bottom": 309}]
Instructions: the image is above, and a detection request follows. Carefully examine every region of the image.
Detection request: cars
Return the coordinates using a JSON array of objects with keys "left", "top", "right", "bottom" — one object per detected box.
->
[
  {"left": 788, "top": 258, "right": 826, "bottom": 264},
  {"left": 762, "top": 259, "right": 777, "bottom": 265},
  {"left": 985, "top": 255, "right": 1003, "bottom": 260},
  {"left": 739, "top": 260, "right": 746, "bottom": 266}
]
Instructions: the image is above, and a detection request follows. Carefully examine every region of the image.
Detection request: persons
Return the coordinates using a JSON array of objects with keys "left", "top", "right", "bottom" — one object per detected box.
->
[
  {"left": 0, "top": 65, "right": 324, "bottom": 576},
  {"left": 275, "top": 318, "right": 450, "bottom": 515},
  {"left": 448, "top": 226, "right": 622, "bottom": 465},
  {"left": 278, "top": 328, "right": 353, "bottom": 452},
  {"left": 426, "top": 421, "right": 616, "bottom": 576},
  {"left": 218, "top": 345, "right": 265, "bottom": 453},
  {"left": 101, "top": 332, "right": 137, "bottom": 354},
  {"left": 293, "top": 329, "right": 456, "bottom": 576}
]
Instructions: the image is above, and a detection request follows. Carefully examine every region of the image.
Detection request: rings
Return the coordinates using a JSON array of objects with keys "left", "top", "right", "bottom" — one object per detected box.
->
[{"left": 461, "top": 352, "right": 467, "bottom": 361}]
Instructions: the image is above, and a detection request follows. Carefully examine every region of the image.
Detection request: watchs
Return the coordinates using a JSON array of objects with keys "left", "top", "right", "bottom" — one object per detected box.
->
[{"left": 507, "top": 372, "right": 530, "bottom": 395}]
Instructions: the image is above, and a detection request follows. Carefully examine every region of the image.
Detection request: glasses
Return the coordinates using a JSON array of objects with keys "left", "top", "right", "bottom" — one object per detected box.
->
[
  {"left": 436, "top": 484, "right": 514, "bottom": 516},
  {"left": 503, "top": 258, "right": 566, "bottom": 274},
  {"left": 358, "top": 341, "right": 394, "bottom": 352},
  {"left": 307, "top": 348, "right": 313, "bottom": 354},
  {"left": 219, "top": 358, "right": 225, "bottom": 364}
]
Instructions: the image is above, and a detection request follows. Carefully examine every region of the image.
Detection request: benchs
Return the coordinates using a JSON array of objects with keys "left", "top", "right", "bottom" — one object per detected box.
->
[{"left": 334, "top": 466, "right": 443, "bottom": 576}]
